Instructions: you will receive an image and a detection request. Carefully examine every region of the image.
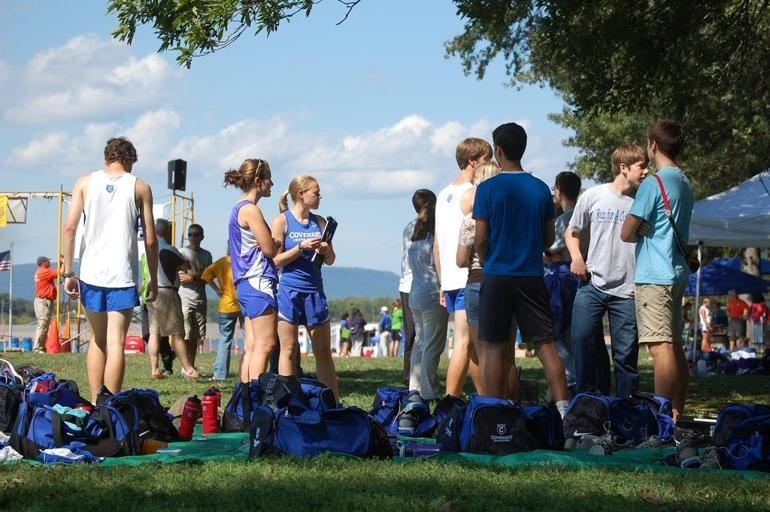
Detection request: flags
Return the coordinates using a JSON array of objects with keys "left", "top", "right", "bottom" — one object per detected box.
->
[{"left": 1, "top": 250, "right": 10, "bottom": 271}]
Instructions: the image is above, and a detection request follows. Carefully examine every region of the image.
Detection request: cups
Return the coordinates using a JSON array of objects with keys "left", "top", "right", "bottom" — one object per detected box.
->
[
  {"left": 589, "top": 445, "right": 612, "bottom": 456},
  {"left": 75, "top": 402, "right": 94, "bottom": 413},
  {"left": 29, "top": 382, "right": 49, "bottom": 393}
]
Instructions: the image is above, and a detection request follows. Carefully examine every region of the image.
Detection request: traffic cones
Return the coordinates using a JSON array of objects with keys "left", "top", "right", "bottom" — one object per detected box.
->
[{"left": 43, "top": 319, "right": 63, "bottom": 353}]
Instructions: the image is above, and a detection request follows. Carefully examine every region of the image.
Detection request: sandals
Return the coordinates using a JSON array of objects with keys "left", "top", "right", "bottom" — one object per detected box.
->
[{"left": 150, "top": 367, "right": 209, "bottom": 382}]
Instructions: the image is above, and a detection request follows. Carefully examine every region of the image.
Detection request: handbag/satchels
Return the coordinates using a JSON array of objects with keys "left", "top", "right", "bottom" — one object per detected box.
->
[
  {"left": 684, "top": 249, "right": 701, "bottom": 271},
  {"left": 0, "top": 359, "right": 79, "bottom": 434},
  {"left": 9, "top": 403, "right": 135, "bottom": 461},
  {"left": 716, "top": 403, "right": 768, "bottom": 471},
  {"left": 370, "top": 387, "right": 434, "bottom": 436},
  {"left": 96, "top": 389, "right": 180, "bottom": 439},
  {"left": 222, "top": 379, "right": 338, "bottom": 432},
  {"left": 433, "top": 394, "right": 563, "bottom": 451},
  {"left": 248, "top": 405, "right": 394, "bottom": 459},
  {"left": 562, "top": 392, "right": 676, "bottom": 438}
]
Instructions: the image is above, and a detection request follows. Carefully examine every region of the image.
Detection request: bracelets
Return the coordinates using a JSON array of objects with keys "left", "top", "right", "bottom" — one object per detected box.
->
[
  {"left": 297, "top": 242, "right": 304, "bottom": 254},
  {"left": 63, "top": 272, "right": 76, "bottom": 278}
]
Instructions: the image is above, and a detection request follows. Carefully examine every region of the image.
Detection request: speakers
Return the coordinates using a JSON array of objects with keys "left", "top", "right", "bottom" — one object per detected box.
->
[{"left": 168, "top": 159, "right": 187, "bottom": 191}]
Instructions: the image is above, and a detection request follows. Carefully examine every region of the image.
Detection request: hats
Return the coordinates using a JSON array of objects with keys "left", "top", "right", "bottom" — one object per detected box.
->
[
  {"left": 37, "top": 256, "right": 51, "bottom": 265},
  {"left": 377, "top": 306, "right": 389, "bottom": 312}
]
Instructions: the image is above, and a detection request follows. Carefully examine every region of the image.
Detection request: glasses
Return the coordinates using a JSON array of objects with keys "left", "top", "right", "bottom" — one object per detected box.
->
[{"left": 188, "top": 232, "right": 202, "bottom": 238}]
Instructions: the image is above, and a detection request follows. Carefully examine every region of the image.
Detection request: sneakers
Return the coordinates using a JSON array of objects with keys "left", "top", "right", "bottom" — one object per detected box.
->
[
  {"left": 580, "top": 430, "right": 634, "bottom": 451},
  {"left": 676, "top": 439, "right": 702, "bottom": 470},
  {"left": 629, "top": 435, "right": 675, "bottom": 450},
  {"left": 702, "top": 446, "right": 725, "bottom": 470},
  {"left": 397, "top": 399, "right": 422, "bottom": 433}
]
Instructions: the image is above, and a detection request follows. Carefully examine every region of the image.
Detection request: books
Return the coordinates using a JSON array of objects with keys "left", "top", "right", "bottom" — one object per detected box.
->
[{"left": 311, "top": 216, "right": 339, "bottom": 268}]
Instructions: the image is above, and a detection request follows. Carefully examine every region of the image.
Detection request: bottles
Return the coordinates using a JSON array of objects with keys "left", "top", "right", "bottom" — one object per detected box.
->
[
  {"left": 395, "top": 440, "right": 445, "bottom": 458},
  {"left": 211, "top": 385, "right": 221, "bottom": 419},
  {"left": 201, "top": 387, "right": 219, "bottom": 433},
  {"left": 697, "top": 358, "right": 707, "bottom": 377},
  {"left": 178, "top": 393, "right": 201, "bottom": 440}
]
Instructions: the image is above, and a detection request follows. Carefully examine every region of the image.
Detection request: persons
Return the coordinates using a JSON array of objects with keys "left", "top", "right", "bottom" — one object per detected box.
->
[
  {"left": 139, "top": 249, "right": 177, "bottom": 379},
  {"left": 141, "top": 218, "right": 201, "bottom": 379},
  {"left": 698, "top": 293, "right": 768, "bottom": 356},
  {"left": 32, "top": 254, "right": 64, "bottom": 353},
  {"left": 337, "top": 299, "right": 404, "bottom": 357},
  {"left": 270, "top": 174, "right": 344, "bottom": 410},
  {"left": 201, "top": 239, "right": 246, "bottom": 381},
  {"left": 223, "top": 158, "right": 278, "bottom": 385},
  {"left": 62, "top": 135, "right": 160, "bottom": 408},
  {"left": 178, "top": 223, "right": 213, "bottom": 379}
]
